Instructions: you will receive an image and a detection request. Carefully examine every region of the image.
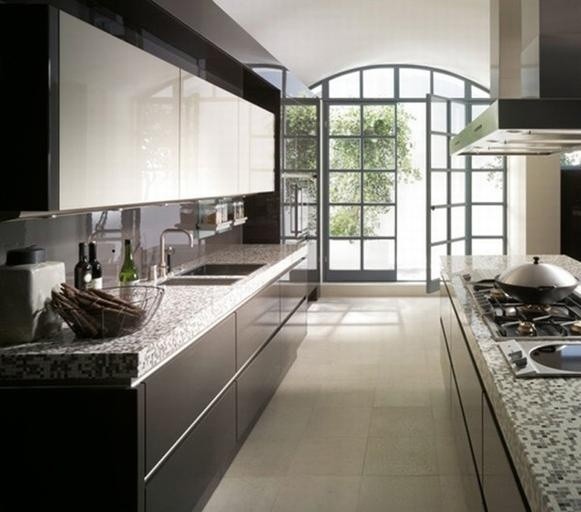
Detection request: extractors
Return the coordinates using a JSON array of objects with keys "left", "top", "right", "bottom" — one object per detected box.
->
[{"left": 445, "top": 0, "right": 581, "bottom": 159}]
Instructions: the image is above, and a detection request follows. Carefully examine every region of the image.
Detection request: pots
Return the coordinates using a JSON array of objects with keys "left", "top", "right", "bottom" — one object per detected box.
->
[{"left": 491, "top": 254, "right": 579, "bottom": 305}]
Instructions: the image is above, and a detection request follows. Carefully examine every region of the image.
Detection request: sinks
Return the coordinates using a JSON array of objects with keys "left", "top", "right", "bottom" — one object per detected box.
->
[
  {"left": 157, "top": 277, "right": 243, "bottom": 286},
  {"left": 174, "top": 262, "right": 267, "bottom": 276}
]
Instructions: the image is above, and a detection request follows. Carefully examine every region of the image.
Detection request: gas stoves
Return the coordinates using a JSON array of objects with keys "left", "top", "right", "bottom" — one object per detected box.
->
[{"left": 462, "top": 273, "right": 581, "bottom": 339}]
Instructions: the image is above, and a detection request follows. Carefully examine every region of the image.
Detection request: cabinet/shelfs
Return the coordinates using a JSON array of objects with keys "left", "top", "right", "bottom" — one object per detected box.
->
[
  {"left": 438, "top": 275, "right": 529, "bottom": 511},
  {"left": 0, "top": 259, "right": 308, "bottom": 511},
  {"left": 180, "top": 69, "right": 239, "bottom": 206},
  {"left": 0, "top": 0, "right": 178, "bottom": 223},
  {"left": 240, "top": 96, "right": 277, "bottom": 196}
]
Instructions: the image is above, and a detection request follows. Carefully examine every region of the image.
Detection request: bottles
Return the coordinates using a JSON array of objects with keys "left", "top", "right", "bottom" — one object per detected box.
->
[
  {"left": 119, "top": 239, "right": 140, "bottom": 306},
  {"left": 74, "top": 241, "right": 93, "bottom": 292},
  {"left": 85, "top": 240, "right": 105, "bottom": 290},
  {"left": 179, "top": 201, "right": 245, "bottom": 227}
]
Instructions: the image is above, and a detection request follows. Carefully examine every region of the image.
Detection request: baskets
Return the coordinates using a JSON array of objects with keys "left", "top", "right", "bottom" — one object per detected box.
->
[{"left": 47, "top": 285, "right": 165, "bottom": 340}]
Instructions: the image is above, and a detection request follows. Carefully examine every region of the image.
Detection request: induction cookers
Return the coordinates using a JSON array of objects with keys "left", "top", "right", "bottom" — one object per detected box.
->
[{"left": 497, "top": 339, "right": 581, "bottom": 378}]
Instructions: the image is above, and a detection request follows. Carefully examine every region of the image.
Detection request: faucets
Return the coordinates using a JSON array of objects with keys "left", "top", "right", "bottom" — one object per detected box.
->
[{"left": 158, "top": 228, "right": 194, "bottom": 276}]
